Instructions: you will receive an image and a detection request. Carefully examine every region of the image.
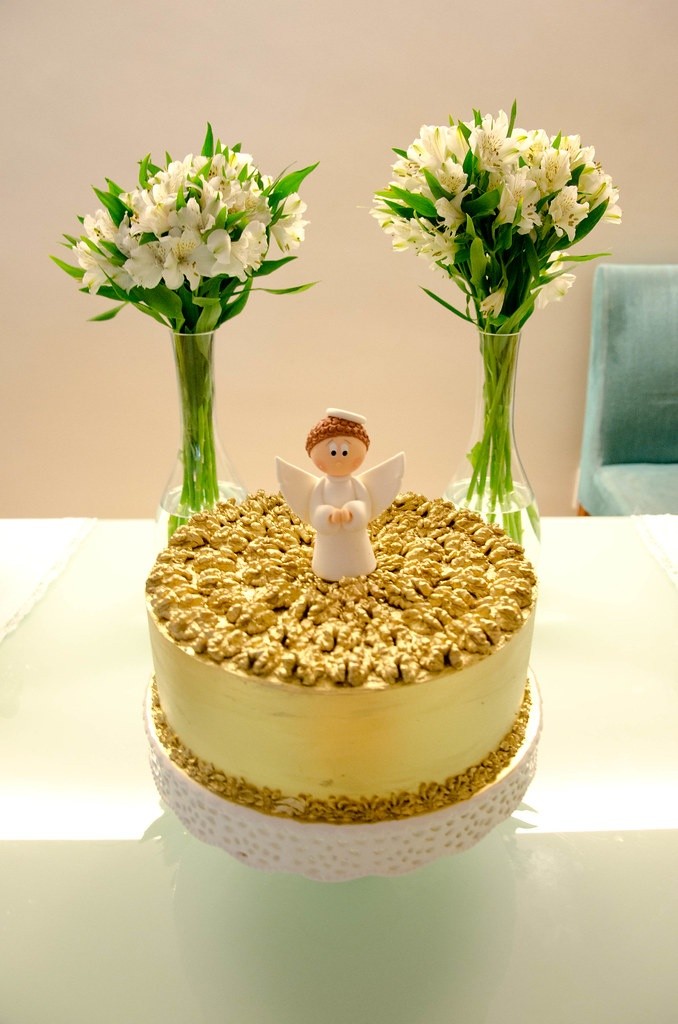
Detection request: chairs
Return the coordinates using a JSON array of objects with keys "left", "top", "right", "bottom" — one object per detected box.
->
[{"left": 573, "top": 263, "right": 678, "bottom": 516}]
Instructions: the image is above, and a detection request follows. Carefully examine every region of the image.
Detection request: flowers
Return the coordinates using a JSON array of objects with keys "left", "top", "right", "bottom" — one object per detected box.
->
[
  {"left": 354, "top": 97, "right": 622, "bottom": 550},
  {"left": 48, "top": 121, "right": 320, "bottom": 538}
]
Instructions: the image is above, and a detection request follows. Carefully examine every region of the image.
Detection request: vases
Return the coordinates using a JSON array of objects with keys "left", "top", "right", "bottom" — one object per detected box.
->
[
  {"left": 154, "top": 327, "right": 248, "bottom": 548},
  {"left": 443, "top": 321, "right": 543, "bottom": 565}
]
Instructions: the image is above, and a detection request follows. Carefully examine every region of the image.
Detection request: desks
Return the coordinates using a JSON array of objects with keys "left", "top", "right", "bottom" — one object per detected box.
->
[{"left": 0, "top": 517, "right": 678, "bottom": 1024}]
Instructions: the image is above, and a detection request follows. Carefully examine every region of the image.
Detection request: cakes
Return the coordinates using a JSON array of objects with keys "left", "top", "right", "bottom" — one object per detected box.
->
[{"left": 143, "top": 408, "right": 539, "bottom": 823}]
